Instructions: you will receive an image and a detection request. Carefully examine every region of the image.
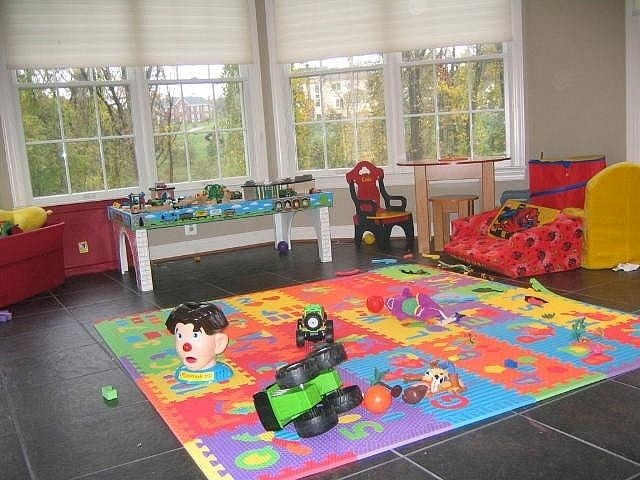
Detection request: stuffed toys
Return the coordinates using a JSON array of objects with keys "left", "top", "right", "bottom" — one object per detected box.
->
[{"left": 0, "top": 206, "right": 53, "bottom": 237}]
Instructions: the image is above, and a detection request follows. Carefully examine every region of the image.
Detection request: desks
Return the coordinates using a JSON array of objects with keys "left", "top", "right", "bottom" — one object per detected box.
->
[
  {"left": 395, "top": 154, "right": 512, "bottom": 256},
  {"left": 109, "top": 190, "right": 334, "bottom": 291}
]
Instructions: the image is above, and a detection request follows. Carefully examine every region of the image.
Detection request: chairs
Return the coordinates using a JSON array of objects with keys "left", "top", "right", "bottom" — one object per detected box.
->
[{"left": 344, "top": 159, "right": 415, "bottom": 251}]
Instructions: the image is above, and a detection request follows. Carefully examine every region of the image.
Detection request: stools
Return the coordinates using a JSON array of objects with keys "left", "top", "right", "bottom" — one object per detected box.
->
[{"left": 427, "top": 193, "right": 479, "bottom": 252}]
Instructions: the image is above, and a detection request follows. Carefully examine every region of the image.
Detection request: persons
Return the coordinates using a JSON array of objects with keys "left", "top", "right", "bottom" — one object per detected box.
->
[{"left": 165, "top": 298, "right": 234, "bottom": 389}]
